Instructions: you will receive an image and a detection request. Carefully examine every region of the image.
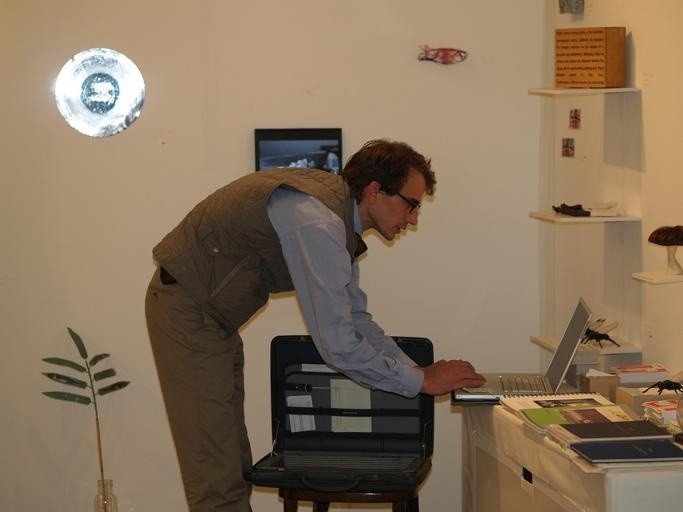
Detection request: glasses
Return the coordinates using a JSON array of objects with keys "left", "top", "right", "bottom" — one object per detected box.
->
[{"left": 397, "top": 191, "right": 421, "bottom": 215}]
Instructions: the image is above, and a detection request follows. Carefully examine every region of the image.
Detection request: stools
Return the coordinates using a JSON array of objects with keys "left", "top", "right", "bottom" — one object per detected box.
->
[{"left": 278, "top": 459, "right": 432, "bottom": 512}]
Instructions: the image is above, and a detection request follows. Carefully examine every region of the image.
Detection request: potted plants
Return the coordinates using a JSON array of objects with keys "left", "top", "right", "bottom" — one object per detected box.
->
[{"left": 39, "top": 322, "right": 131, "bottom": 512}]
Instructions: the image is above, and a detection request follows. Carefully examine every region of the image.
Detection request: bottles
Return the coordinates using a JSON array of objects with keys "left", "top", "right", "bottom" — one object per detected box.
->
[{"left": 92, "top": 478, "right": 117, "bottom": 511}]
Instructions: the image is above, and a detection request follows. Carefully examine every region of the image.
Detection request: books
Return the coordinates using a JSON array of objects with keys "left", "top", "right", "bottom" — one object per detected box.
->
[
  {"left": 571, "top": 342, "right": 683, "bottom": 417},
  {"left": 498, "top": 392, "right": 681, "bottom": 466},
  {"left": 582, "top": 201, "right": 625, "bottom": 217}
]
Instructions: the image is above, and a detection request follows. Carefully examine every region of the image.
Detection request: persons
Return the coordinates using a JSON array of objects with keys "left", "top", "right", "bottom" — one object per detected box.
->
[{"left": 145, "top": 139, "right": 486, "bottom": 512}]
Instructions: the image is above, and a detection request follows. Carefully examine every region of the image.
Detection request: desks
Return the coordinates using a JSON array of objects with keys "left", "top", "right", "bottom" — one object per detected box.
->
[{"left": 458, "top": 394, "right": 681, "bottom": 511}]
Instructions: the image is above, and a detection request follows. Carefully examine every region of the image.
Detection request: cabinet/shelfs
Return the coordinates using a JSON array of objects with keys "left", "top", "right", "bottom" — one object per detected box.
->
[{"left": 525, "top": 86, "right": 642, "bottom": 355}]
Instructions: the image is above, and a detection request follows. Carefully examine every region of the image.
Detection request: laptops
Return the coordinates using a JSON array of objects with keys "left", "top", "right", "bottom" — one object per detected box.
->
[{"left": 450, "top": 295, "right": 593, "bottom": 403}]
